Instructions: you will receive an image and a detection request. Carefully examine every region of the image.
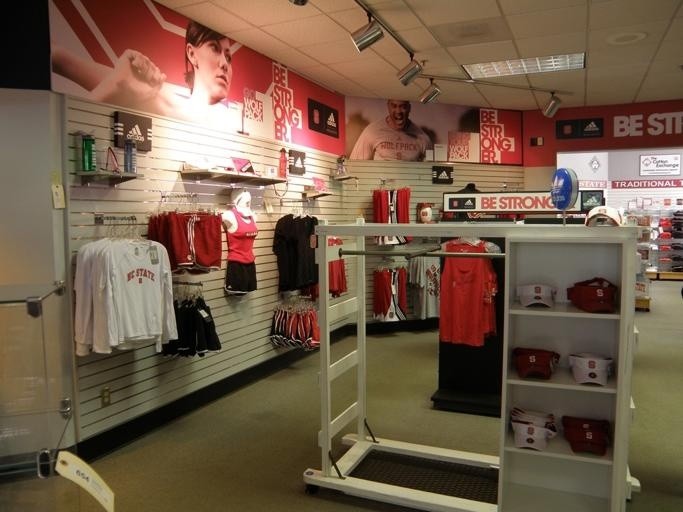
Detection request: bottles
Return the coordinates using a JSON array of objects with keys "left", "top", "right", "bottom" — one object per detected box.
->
[
  {"left": 123, "top": 140, "right": 136, "bottom": 172},
  {"left": 278, "top": 148, "right": 287, "bottom": 178},
  {"left": 81, "top": 135, "right": 96, "bottom": 171}
]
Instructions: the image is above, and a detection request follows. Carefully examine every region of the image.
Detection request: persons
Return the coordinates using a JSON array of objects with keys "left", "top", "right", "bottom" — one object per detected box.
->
[
  {"left": 348, "top": 100, "right": 433, "bottom": 164},
  {"left": 219, "top": 189, "right": 258, "bottom": 296},
  {"left": 51, "top": 20, "right": 240, "bottom": 130},
  {"left": 83, "top": 48, "right": 167, "bottom": 114}
]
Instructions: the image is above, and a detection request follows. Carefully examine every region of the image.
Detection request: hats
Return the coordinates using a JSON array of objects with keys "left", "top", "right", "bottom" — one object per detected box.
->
[
  {"left": 584, "top": 204, "right": 623, "bottom": 228},
  {"left": 560, "top": 412, "right": 611, "bottom": 455},
  {"left": 567, "top": 349, "right": 614, "bottom": 386},
  {"left": 511, "top": 283, "right": 559, "bottom": 307},
  {"left": 229, "top": 188, "right": 253, "bottom": 218},
  {"left": 566, "top": 276, "right": 620, "bottom": 314},
  {"left": 510, "top": 345, "right": 561, "bottom": 379},
  {"left": 508, "top": 402, "right": 559, "bottom": 452}
]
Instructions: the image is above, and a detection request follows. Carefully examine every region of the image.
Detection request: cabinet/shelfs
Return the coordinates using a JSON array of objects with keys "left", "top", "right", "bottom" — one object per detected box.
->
[{"left": 302, "top": 216, "right": 641, "bottom": 512}]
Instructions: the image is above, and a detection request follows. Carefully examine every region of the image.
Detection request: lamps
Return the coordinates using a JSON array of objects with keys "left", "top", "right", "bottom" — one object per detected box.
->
[{"left": 350, "top": 10, "right": 563, "bottom": 119}]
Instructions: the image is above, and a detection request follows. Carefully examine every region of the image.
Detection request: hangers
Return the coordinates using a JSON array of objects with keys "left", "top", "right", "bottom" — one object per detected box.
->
[
  {"left": 154, "top": 191, "right": 220, "bottom": 217},
  {"left": 103, "top": 214, "right": 151, "bottom": 246}
]
